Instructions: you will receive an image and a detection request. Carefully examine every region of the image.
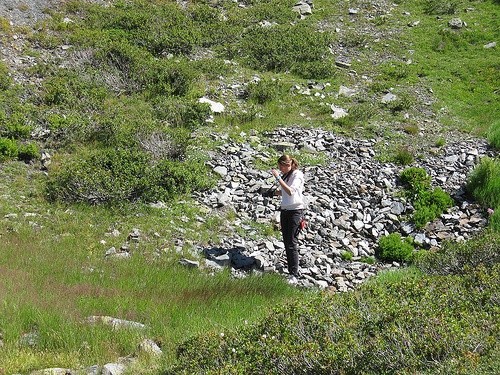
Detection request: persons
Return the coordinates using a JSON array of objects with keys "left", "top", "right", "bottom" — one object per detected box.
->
[{"left": 266, "top": 154, "right": 306, "bottom": 284}]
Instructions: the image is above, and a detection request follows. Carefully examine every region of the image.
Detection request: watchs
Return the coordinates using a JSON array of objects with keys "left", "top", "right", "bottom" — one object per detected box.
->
[{"left": 275, "top": 175, "right": 281, "bottom": 180}]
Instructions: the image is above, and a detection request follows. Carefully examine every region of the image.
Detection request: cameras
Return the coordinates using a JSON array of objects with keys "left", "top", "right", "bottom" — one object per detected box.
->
[{"left": 262, "top": 191, "right": 273, "bottom": 198}]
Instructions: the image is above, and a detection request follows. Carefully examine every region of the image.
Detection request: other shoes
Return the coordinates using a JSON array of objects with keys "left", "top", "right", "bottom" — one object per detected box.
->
[{"left": 281, "top": 275, "right": 298, "bottom": 286}]
